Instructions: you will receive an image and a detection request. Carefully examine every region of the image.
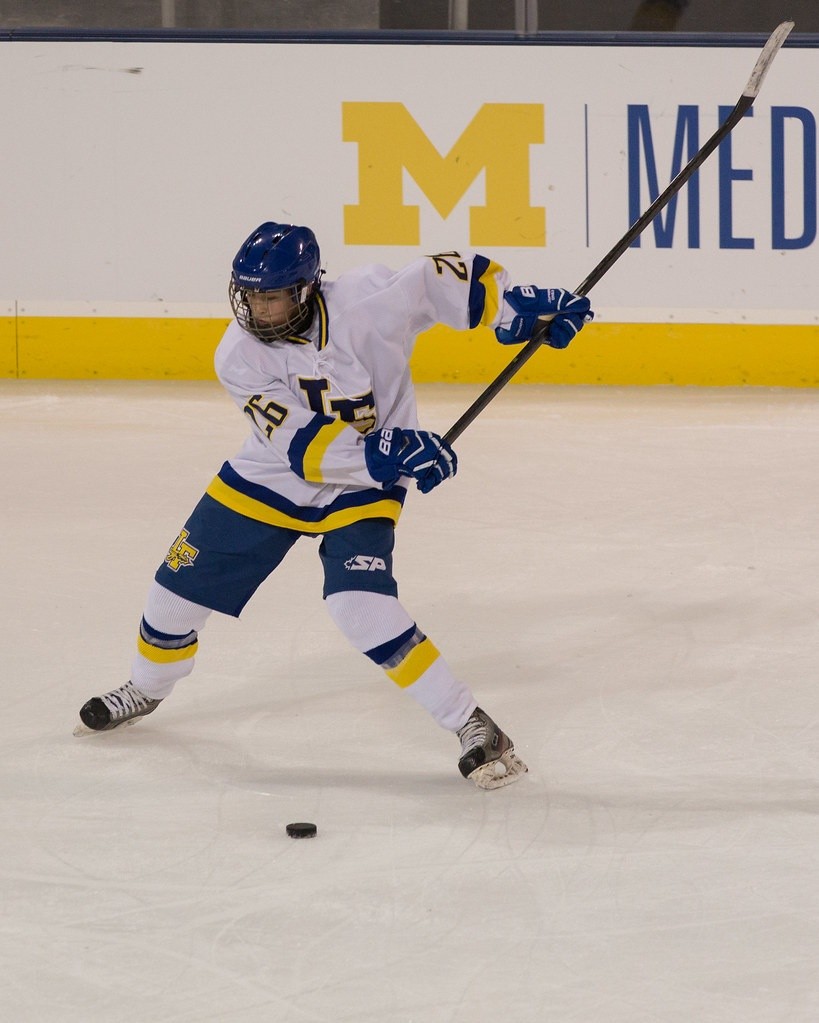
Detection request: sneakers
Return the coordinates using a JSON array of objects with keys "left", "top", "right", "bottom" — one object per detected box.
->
[
  {"left": 71, "top": 681, "right": 166, "bottom": 736},
  {"left": 457, "top": 707, "right": 529, "bottom": 791}
]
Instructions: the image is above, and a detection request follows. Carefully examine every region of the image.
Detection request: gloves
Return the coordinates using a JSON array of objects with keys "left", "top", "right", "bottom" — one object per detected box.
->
[
  {"left": 495, "top": 285, "right": 594, "bottom": 350},
  {"left": 364, "top": 428, "right": 458, "bottom": 494}
]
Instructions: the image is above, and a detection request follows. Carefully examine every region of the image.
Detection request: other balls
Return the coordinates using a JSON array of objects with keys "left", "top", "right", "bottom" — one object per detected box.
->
[{"left": 285, "top": 821, "right": 317, "bottom": 840}]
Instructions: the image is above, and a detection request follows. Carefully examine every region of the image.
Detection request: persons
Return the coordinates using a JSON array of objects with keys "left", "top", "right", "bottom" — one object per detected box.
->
[{"left": 73, "top": 219, "right": 596, "bottom": 791}]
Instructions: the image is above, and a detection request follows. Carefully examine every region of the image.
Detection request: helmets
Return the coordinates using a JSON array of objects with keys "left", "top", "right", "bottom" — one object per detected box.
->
[{"left": 229, "top": 221, "right": 325, "bottom": 344}]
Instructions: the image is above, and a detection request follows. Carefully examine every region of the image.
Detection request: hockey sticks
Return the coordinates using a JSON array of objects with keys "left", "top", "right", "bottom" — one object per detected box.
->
[{"left": 437, "top": 16, "right": 802, "bottom": 451}]
